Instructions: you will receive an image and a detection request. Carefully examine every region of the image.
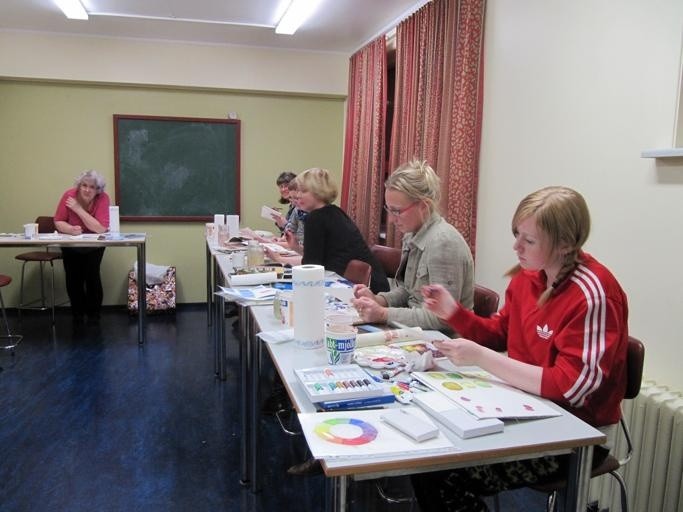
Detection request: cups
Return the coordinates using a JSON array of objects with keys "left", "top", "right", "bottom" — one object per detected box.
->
[
  {"left": 232, "top": 253, "right": 245, "bottom": 268},
  {"left": 273, "top": 290, "right": 289, "bottom": 324},
  {"left": 24, "top": 224, "right": 33, "bottom": 239},
  {"left": 33, "top": 224, "right": 38, "bottom": 236},
  {"left": 326, "top": 325, "right": 359, "bottom": 364},
  {"left": 325, "top": 315, "right": 353, "bottom": 328}
]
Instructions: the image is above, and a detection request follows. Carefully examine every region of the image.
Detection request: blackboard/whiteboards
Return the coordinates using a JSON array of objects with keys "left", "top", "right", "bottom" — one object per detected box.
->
[{"left": 113, "top": 115, "right": 240, "bottom": 223}]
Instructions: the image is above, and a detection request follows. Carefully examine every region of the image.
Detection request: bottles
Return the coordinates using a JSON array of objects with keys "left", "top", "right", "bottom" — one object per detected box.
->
[
  {"left": 214, "top": 214, "right": 224, "bottom": 231},
  {"left": 218, "top": 225, "right": 229, "bottom": 245},
  {"left": 205, "top": 223, "right": 217, "bottom": 237},
  {"left": 226, "top": 214, "right": 239, "bottom": 235},
  {"left": 248, "top": 244, "right": 264, "bottom": 267}
]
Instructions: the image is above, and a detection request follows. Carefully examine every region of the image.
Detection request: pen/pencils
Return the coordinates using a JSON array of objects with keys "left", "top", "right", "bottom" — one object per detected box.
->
[{"left": 316, "top": 406, "right": 388, "bottom": 411}]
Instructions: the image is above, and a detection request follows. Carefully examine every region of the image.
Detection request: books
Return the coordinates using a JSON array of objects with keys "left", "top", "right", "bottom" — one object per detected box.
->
[
  {"left": 352, "top": 325, "right": 448, "bottom": 369},
  {"left": 261, "top": 206, "right": 281, "bottom": 222}
]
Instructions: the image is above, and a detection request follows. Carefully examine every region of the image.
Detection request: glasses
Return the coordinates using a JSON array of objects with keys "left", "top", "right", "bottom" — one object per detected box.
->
[{"left": 384, "top": 202, "right": 417, "bottom": 216}]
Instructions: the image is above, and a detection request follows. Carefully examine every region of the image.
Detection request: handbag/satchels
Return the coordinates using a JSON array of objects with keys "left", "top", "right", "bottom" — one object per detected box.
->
[{"left": 128, "top": 267, "right": 177, "bottom": 316}]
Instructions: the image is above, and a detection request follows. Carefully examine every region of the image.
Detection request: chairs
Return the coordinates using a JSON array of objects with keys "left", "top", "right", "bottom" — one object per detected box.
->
[
  {"left": 374, "top": 286, "right": 500, "bottom": 503},
  {"left": 275, "top": 259, "right": 371, "bottom": 436},
  {"left": 0, "top": 274, "right": 23, "bottom": 355},
  {"left": 14, "top": 216, "right": 69, "bottom": 326},
  {"left": 495, "top": 336, "right": 644, "bottom": 512},
  {"left": 370, "top": 243, "right": 402, "bottom": 279}
]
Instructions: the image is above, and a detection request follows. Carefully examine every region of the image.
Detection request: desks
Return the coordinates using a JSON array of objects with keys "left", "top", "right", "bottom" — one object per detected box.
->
[
  {"left": 0, "top": 232, "right": 148, "bottom": 345},
  {"left": 213, "top": 251, "right": 356, "bottom": 486},
  {"left": 246, "top": 302, "right": 607, "bottom": 511},
  {"left": 204, "top": 232, "right": 284, "bottom": 377}
]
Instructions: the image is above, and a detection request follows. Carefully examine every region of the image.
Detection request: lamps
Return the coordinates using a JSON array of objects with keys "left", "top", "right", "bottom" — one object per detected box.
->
[
  {"left": 53, "top": 0, "right": 88, "bottom": 21},
  {"left": 274, "top": 0, "right": 315, "bottom": 35}
]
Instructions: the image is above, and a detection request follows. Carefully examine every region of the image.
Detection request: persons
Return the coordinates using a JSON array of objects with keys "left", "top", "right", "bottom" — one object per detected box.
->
[
  {"left": 349, "top": 161, "right": 474, "bottom": 337},
  {"left": 271, "top": 176, "right": 307, "bottom": 256},
  {"left": 54, "top": 168, "right": 111, "bottom": 329},
  {"left": 263, "top": 169, "right": 391, "bottom": 295},
  {"left": 413, "top": 185, "right": 627, "bottom": 512},
  {"left": 222, "top": 171, "right": 297, "bottom": 319}
]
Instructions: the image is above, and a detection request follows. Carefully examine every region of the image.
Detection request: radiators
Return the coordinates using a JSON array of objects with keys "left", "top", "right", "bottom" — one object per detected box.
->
[{"left": 585, "top": 383, "right": 683, "bottom": 512}]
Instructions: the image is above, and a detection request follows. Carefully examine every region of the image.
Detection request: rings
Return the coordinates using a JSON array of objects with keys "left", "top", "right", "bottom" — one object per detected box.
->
[{"left": 357, "top": 307, "right": 361, "bottom": 313}]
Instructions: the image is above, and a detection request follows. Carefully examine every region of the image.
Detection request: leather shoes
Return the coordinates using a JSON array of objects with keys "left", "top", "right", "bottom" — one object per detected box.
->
[{"left": 287, "top": 458, "right": 324, "bottom": 476}]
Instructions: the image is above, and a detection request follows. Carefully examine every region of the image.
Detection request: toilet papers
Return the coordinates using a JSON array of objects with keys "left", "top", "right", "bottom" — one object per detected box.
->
[
  {"left": 108, "top": 205, "right": 120, "bottom": 236},
  {"left": 214, "top": 213, "right": 227, "bottom": 243},
  {"left": 226, "top": 215, "right": 240, "bottom": 242},
  {"left": 291, "top": 263, "right": 327, "bottom": 349},
  {"left": 231, "top": 273, "right": 279, "bottom": 285}
]
